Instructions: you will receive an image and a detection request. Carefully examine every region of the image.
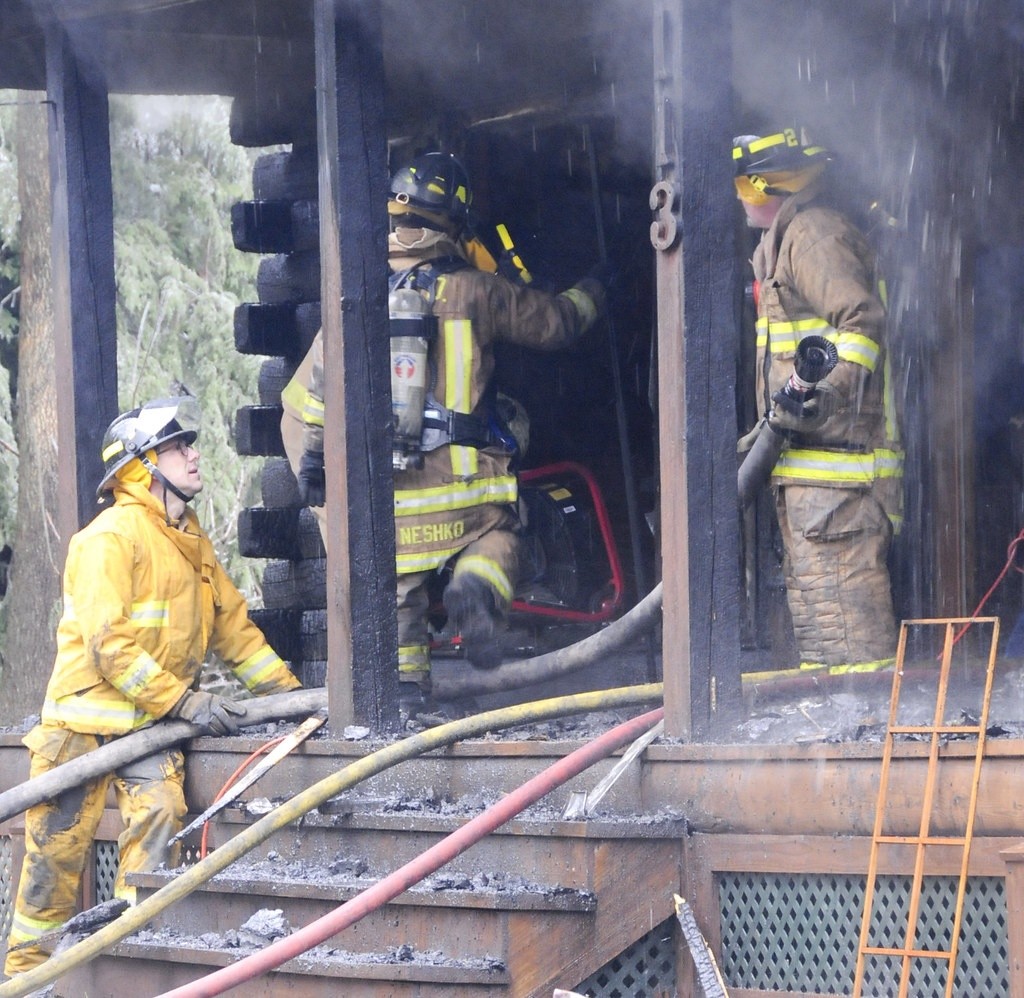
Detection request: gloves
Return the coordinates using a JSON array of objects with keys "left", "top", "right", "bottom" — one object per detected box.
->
[
  {"left": 167, "top": 688, "right": 246, "bottom": 737},
  {"left": 587, "top": 258, "right": 629, "bottom": 302},
  {"left": 297, "top": 450, "right": 326, "bottom": 508},
  {"left": 768, "top": 379, "right": 851, "bottom": 432}
]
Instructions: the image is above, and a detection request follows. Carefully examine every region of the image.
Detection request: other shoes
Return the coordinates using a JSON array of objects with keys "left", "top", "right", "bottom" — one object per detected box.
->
[
  {"left": 441, "top": 574, "right": 508, "bottom": 672},
  {"left": 395, "top": 684, "right": 436, "bottom": 716}
]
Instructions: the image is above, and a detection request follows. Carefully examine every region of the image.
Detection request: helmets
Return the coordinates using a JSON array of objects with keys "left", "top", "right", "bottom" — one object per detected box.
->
[
  {"left": 96, "top": 405, "right": 198, "bottom": 500},
  {"left": 734, "top": 102, "right": 840, "bottom": 176},
  {"left": 388, "top": 151, "right": 476, "bottom": 222}
]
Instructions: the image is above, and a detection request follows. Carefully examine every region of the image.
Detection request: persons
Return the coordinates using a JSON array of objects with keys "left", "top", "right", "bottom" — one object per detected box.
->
[
  {"left": 281, "top": 152, "right": 611, "bottom": 697},
  {"left": 3, "top": 397, "right": 304, "bottom": 998},
  {"left": 734, "top": 125, "right": 904, "bottom": 667}
]
